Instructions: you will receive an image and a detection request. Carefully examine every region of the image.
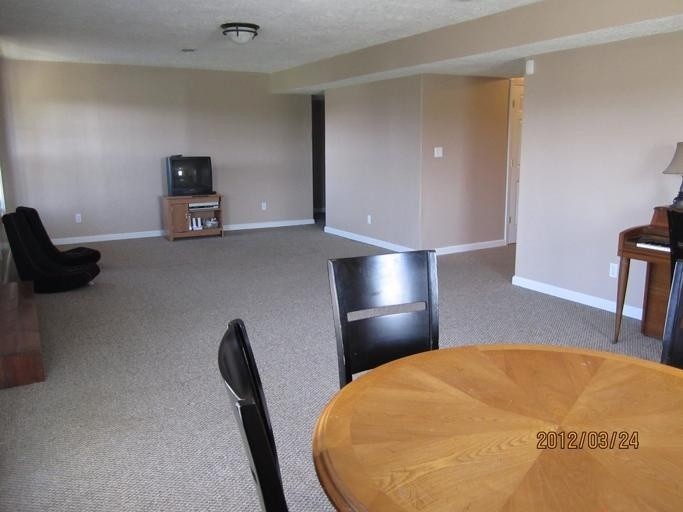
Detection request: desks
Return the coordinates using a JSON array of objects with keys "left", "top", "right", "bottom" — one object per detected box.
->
[{"left": 309, "top": 344, "right": 681, "bottom": 511}]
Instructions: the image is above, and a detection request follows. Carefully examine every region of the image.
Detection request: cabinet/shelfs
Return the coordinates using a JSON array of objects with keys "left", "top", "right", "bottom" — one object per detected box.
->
[{"left": 158, "top": 192, "right": 224, "bottom": 242}]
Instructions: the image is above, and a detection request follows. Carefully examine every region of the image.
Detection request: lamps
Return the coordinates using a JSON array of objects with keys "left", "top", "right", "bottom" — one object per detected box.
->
[
  {"left": 221, "top": 22, "right": 259, "bottom": 46},
  {"left": 658, "top": 137, "right": 683, "bottom": 206}
]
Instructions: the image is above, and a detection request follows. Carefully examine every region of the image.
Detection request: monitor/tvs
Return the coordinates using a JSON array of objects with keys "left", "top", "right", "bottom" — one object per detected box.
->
[{"left": 166, "top": 156, "right": 212, "bottom": 197}]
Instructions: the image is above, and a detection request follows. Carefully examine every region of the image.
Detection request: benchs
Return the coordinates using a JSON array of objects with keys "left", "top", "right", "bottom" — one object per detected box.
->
[{"left": 0, "top": 278, "right": 43, "bottom": 390}]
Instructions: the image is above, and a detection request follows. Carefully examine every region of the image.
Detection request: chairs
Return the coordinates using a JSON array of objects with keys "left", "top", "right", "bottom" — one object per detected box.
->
[
  {"left": 16, "top": 206, "right": 100, "bottom": 262},
  {"left": 216, "top": 312, "right": 295, "bottom": 512},
  {"left": 659, "top": 258, "right": 683, "bottom": 370},
  {"left": 1, "top": 211, "right": 100, "bottom": 295},
  {"left": 325, "top": 250, "right": 440, "bottom": 390}
]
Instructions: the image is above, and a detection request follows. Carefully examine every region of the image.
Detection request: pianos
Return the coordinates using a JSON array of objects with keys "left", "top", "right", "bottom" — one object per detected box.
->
[{"left": 612, "top": 206, "right": 671, "bottom": 344}]
